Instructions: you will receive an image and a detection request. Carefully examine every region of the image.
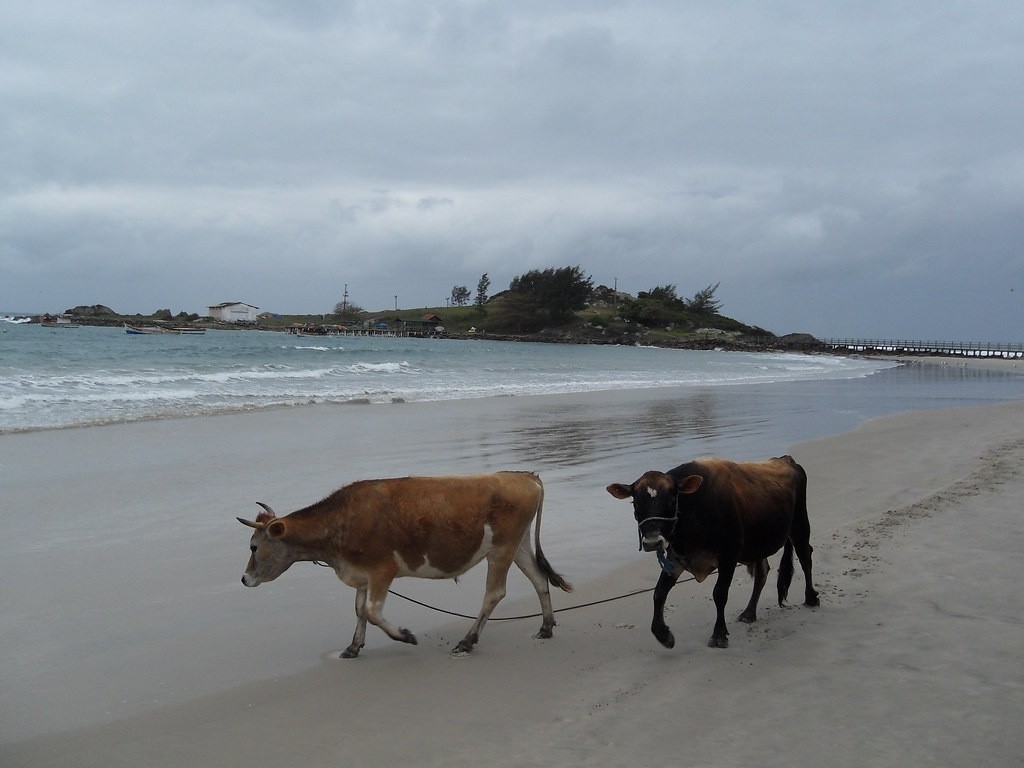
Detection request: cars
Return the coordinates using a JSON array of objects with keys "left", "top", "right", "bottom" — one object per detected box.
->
[{"left": 376, "top": 323, "right": 388, "bottom": 330}]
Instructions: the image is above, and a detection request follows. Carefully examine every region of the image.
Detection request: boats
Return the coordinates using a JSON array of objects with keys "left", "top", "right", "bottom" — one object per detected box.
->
[
  {"left": 39, "top": 316, "right": 80, "bottom": 328},
  {"left": 123, "top": 321, "right": 207, "bottom": 335}
]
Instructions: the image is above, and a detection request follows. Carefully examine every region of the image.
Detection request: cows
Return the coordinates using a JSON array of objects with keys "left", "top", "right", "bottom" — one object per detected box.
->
[
  {"left": 236, "top": 469, "right": 574, "bottom": 659},
  {"left": 606, "top": 454, "right": 820, "bottom": 650}
]
